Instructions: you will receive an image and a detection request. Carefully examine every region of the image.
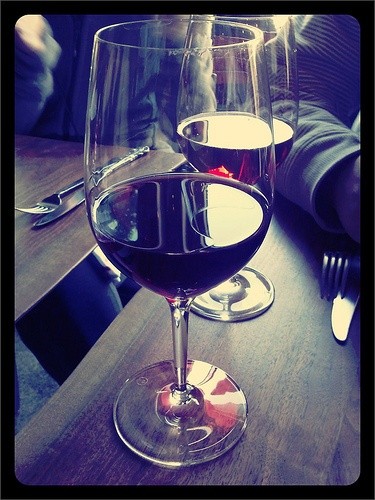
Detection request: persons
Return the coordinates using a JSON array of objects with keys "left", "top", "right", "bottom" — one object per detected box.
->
[
  {"left": 234, "top": 14, "right": 360, "bottom": 242},
  {"left": 15, "top": 13, "right": 219, "bottom": 154}
]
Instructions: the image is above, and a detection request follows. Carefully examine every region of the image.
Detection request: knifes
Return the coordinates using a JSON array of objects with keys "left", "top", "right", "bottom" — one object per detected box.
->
[
  {"left": 332, "top": 253, "right": 360, "bottom": 343},
  {"left": 31, "top": 146, "right": 150, "bottom": 228}
]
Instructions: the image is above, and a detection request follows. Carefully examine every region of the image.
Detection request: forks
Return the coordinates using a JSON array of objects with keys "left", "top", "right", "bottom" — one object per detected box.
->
[
  {"left": 16, "top": 157, "right": 119, "bottom": 214},
  {"left": 321, "top": 250, "right": 349, "bottom": 301}
]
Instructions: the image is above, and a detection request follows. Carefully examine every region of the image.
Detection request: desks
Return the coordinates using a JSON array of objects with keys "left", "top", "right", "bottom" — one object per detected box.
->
[
  {"left": 15, "top": 182, "right": 361, "bottom": 487},
  {"left": 14, "top": 134, "right": 187, "bottom": 322}
]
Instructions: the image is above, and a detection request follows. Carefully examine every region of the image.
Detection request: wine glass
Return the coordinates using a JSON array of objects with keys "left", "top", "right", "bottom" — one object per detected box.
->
[
  {"left": 83, "top": 20, "right": 276, "bottom": 470},
  {"left": 176, "top": 14, "right": 299, "bottom": 323}
]
